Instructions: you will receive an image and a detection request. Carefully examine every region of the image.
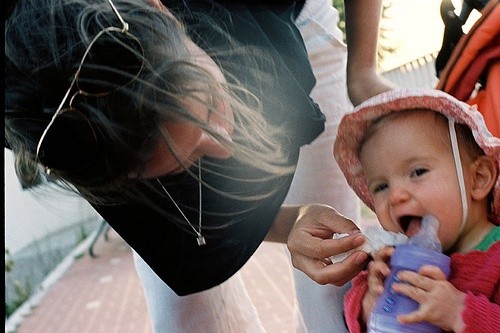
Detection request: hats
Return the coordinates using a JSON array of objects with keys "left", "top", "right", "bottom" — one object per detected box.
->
[{"left": 333, "top": 85, "right": 500, "bottom": 223}]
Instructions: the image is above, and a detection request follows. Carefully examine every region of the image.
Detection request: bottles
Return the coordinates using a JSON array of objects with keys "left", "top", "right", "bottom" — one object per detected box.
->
[{"left": 367, "top": 215, "right": 451, "bottom": 333}]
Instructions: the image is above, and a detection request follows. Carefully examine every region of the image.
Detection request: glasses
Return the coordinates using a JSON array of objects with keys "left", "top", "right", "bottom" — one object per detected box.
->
[{"left": 33, "top": 0, "right": 146, "bottom": 172}]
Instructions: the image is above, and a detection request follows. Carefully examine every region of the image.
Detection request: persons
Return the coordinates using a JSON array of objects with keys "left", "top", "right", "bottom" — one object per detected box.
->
[
  {"left": 456, "top": 0, "right": 493, "bottom": 25},
  {"left": 330, "top": 87, "right": 500, "bottom": 333},
  {"left": 434, "top": 1, "right": 468, "bottom": 80},
  {"left": 0, "top": 1, "right": 399, "bottom": 333}
]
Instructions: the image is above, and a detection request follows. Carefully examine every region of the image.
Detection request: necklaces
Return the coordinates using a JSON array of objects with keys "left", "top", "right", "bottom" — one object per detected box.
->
[{"left": 155, "top": 157, "right": 206, "bottom": 247}]
